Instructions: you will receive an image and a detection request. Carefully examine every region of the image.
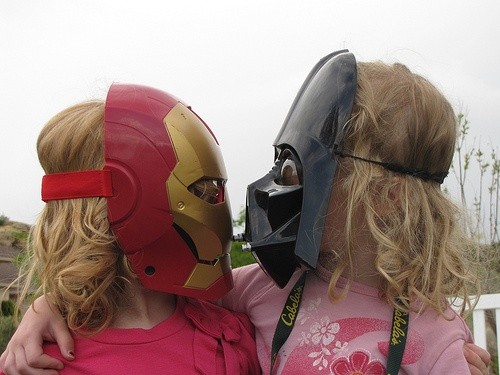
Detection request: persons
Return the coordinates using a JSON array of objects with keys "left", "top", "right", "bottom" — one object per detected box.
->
[
  {"left": 0, "top": 50, "right": 476, "bottom": 375},
  {"left": 1, "top": 83, "right": 490, "bottom": 375}
]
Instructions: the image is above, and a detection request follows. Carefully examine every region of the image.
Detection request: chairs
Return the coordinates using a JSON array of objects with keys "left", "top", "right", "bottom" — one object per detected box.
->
[{"left": 445, "top": 294, "right": 500, "bottom": 375}]
